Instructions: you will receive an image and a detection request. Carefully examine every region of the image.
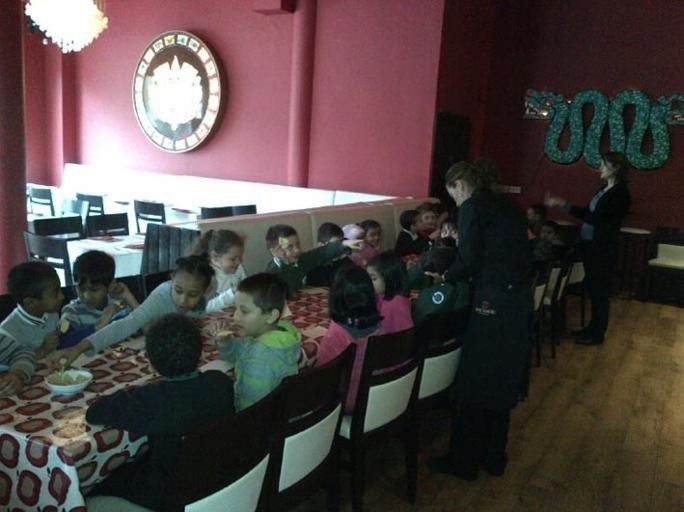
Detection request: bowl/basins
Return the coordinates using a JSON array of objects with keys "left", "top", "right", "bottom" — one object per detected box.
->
[{"left": 45, "top": 370, "right": 94, "bottom": 396}]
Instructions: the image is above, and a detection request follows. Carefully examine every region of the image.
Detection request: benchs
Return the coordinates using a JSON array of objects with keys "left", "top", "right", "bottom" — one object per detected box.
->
[{"left": 140, "top": 185, "right": 440, "bottom": 278}]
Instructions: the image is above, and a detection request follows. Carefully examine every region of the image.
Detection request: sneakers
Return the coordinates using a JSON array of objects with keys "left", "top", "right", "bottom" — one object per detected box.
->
[
  {"left": 428, "top": 455, "right": 480, "bottom": 485},
  {"left": 488, "top": 453, "right": 504, "bottom": 478},
  {"left": 575, "top": 335, "right": 603, "bottom": 346},
  {"left": 571, "top": 328, "right": 592, "bottom": 337}
]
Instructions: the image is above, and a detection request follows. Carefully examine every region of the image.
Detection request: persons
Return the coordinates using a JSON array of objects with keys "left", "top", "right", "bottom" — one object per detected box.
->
[{"left": 543, "top": 151, "right": 632, "bottom": 347}]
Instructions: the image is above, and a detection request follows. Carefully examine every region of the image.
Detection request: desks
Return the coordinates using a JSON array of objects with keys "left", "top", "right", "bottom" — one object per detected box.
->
[{"left": 2, "top": 286, "right": 333, "bottom": 510}]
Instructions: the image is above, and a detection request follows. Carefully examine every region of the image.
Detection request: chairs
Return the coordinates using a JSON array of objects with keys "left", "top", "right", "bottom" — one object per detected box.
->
[
  {"left": 344, "top": 303, "right": 471, "bottom": 512},
  {"left": 86, "top": 342, "right": 358, "bottom": 510},
  {"left": 22, "top": 188, "right": 257, "bottom": 308},
  {"left": 535, "top": 263, "right": 575, "bottom": 366}
]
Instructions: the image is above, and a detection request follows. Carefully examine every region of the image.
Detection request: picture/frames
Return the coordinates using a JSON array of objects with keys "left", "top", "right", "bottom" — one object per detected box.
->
[{"left": 132, "top": 29, "right": 225, "bottom": 154}]
